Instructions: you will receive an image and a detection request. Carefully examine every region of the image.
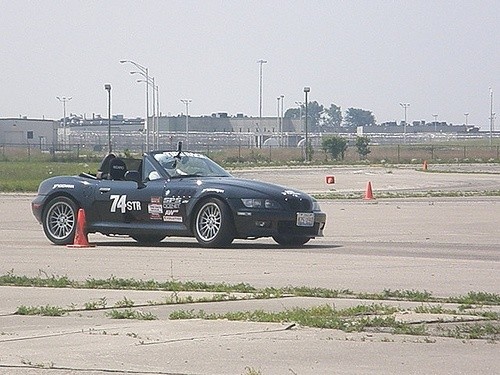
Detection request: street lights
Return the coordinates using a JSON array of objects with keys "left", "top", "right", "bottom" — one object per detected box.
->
[
  {"left": 119, "top": 59, "right": 160, "bottom": 153},
  {"left": 432, "top": 114, "right": 438, "bottom": 135},
  {"left": 180, "top": 98, "right": 193, "bottom": 150},
  {"left": 488, "top": 87, "right": 494, "bottom": 150},
  {"left": 464, "top": 113, "right": 469, "bottom": 134},
  {"left": 280, "top": 95, "right": 284, "bottom": 147},
  {"left": 400, "top": 102, "right": 411, "bottom": 143},
  {"left": 104, "top": 84, "right": 112, "bottom": 153},
  {"left": 55, "top": 96, "right": 73, "bottom": 150},
  {"left": 255, "top": 59, "right": 268, "bottom": 150},
  {"left": 303, "top": 86, "right": 310, "bottom": 162},
  {"left": 277, "top": 97, "right": 281, "bottom": 147}
]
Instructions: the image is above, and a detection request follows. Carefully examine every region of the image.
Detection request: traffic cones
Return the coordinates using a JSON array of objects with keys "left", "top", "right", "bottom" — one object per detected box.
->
[
  {"left": 422, "top": 160, "right": 428, "bottom": 171},
  {"left": 362, "top": 181, "right": 377, "bottom": 200},
  {"left": 65, "top": 209, "right": 97, "bottom": 248},
  {"left": 326, "top": 176, "right": 335, "bottom": 184}
]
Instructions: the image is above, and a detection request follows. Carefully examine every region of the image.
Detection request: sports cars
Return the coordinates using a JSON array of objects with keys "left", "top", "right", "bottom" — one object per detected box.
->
[{"left": 30, "top": 149, "right": 327, "bottom": 250}]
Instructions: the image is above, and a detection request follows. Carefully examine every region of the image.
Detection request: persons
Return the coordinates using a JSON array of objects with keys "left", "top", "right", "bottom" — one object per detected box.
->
[{"left": 149, "top": 154, "right": 187, "bottom": 180}]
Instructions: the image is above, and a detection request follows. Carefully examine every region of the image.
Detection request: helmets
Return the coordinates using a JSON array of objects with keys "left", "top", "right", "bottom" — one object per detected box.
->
[{"left": 154, "top": 154, "right": 178, "bottom": 176}]
